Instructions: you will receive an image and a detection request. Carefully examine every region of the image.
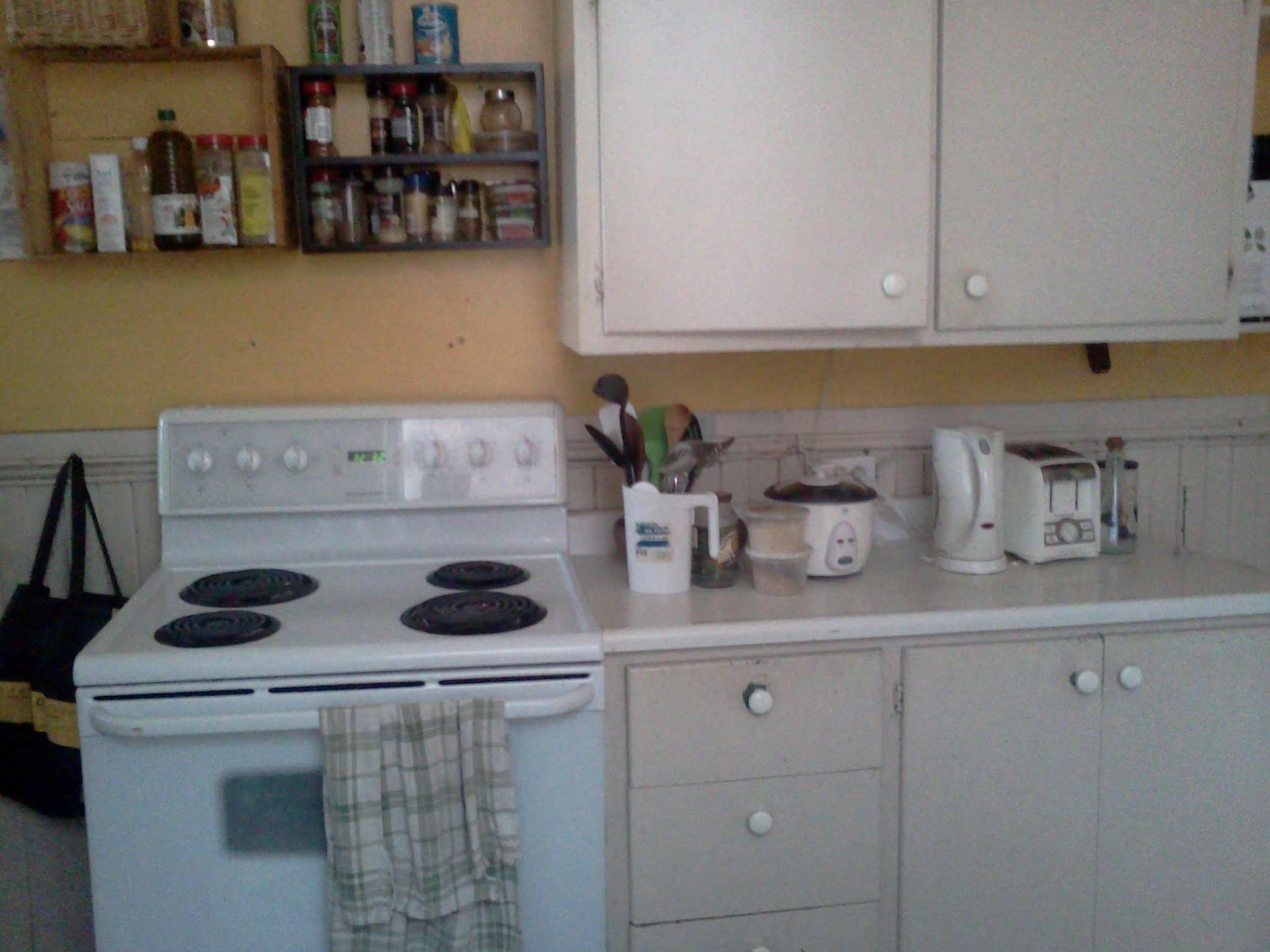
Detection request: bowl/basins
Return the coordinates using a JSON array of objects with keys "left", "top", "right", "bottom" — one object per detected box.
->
[
  {"left": 739, "top": 502, "right": 811, "bottom": 555},
  {"left": 744, "top": 544, "right": 813, "bottom": 595}
]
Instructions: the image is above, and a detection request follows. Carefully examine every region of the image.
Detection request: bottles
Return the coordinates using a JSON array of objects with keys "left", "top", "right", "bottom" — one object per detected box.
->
[
  {"left": 1103, "top": 432, "right": 1135, "bottom": 555},
  {"left": 289, "top": 2, "right": 532, "bottom": 243},
  {"left": 192, "top": 130, "right": 239, "bottom": 248},
  {"left": 177, "top": 1, "right": 239, "bottom": 47},
  {"left": 123, "top": 132, "right": 155, "bottom": 251},
  {"left": 232, "top": 131, "right": 276, "bottom": 247},
  {"left": 146, "top": 103, "right": 202, "bottom": 249}
]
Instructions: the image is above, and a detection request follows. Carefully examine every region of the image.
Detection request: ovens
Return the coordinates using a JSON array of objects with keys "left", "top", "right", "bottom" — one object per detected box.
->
[{"left": 62, "top": 692, "right": 610, "bottom": 950}]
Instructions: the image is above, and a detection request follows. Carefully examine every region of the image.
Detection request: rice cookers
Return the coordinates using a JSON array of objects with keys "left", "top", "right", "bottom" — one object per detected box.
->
[{"left": 761, "top": 463, "right": 878, "bottom": 580}]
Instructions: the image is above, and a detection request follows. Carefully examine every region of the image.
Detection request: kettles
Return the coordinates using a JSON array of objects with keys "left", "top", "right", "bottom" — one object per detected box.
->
[{"left": 919, "top": 415, "right": 1014, "bottom": 574}]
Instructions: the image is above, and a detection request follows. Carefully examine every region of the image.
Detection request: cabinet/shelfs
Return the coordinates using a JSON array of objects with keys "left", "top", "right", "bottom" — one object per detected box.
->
[
  {"left": 554, "top": 0, "right": 1260, "bottom": 356},
  {"left": 282, "top": 59, "right": 549, "bottom": 256},
  {"left": 891, "top": 612, "right": 1270, "bottom": 952},
  {"left": 605, "top": 639, "right": 892, "bottom": 951},
  {"left": 1, "top": 40, "right": 297, "bottom": 259}
]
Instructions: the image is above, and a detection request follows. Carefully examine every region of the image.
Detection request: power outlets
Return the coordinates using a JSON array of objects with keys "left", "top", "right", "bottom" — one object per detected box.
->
[{"left": 826, "top": 454, "right": 875, "bottom": 489}]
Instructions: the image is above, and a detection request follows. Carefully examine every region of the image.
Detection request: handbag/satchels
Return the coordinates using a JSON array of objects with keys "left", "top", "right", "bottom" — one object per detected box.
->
[{"left": 1, "top": 453, "right": 132, "bottom": 819}]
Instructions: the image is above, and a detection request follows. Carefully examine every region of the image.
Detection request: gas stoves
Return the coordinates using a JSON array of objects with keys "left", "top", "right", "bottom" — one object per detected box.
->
[{"left": 66, "top": 399, "right": 607, "bottom": 684}]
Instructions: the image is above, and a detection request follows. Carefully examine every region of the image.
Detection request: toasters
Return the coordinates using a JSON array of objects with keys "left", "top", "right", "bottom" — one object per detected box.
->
[{"left": 999, "top": 440, "right": 1106, "bottom": 564}]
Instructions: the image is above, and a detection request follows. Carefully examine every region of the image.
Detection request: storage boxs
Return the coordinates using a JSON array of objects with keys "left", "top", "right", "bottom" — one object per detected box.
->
[
  {"left": 1, "top": 0, "right": 168, "bottom": 46},
  {"left": 87, "top": 149, "right": 133, "bottom": 254}
]
